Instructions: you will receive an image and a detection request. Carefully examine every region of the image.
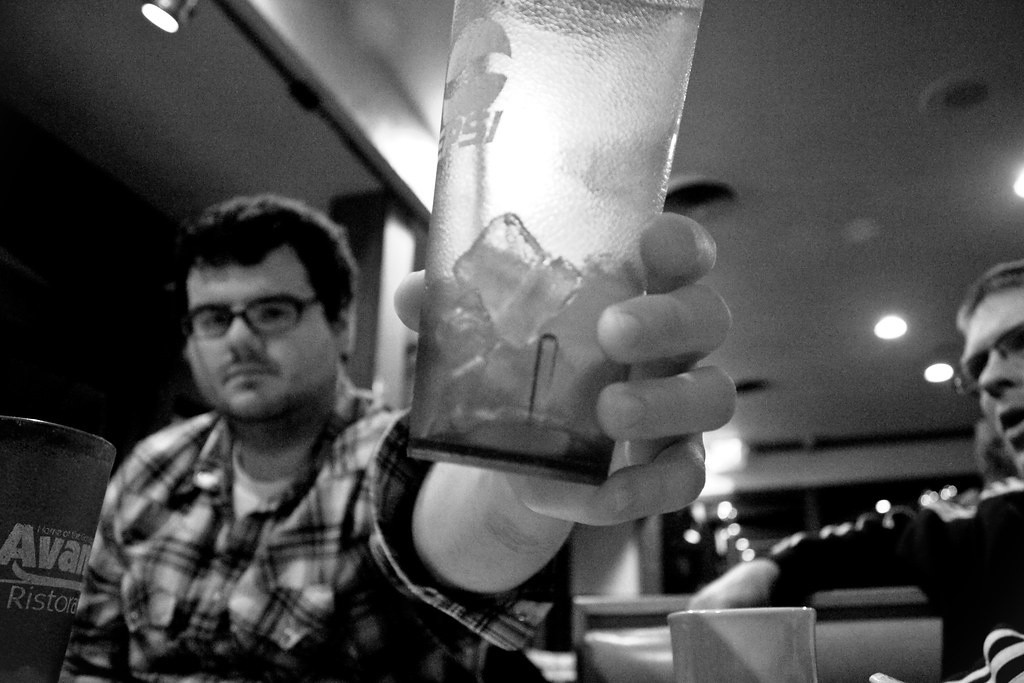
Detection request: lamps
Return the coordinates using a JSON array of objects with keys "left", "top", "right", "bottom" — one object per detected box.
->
[{"left": 139, "top": 1, "right": 198, "bottom": 33}]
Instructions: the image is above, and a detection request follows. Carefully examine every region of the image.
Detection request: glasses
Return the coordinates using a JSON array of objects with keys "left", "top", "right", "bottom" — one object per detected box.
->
[
  {"left": 180, "top": 295, "right": 318, "bottom": 338},
  {"left": 957, "top": 329, "right": 1024, "bottom": 397}
]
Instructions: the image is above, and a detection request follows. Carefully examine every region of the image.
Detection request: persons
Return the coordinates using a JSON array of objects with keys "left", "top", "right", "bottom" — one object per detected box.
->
[
  {"left": 686, "top": 260, "right": 1024, "bottom": 683},
  {"left": 61, "top": 196, "right": 735, "bottom": 683}
]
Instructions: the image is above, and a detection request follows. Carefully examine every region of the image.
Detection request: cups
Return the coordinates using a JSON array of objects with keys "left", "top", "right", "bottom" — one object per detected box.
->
[
  {"left": 0, "top": 414, "right": 117, "bottom": 683},
  {"left": 406, "top": 1, "right": 705, "bottom": 485},
  {"left": 667, "top": 608, "right": 817, "bottom": 683}
]
